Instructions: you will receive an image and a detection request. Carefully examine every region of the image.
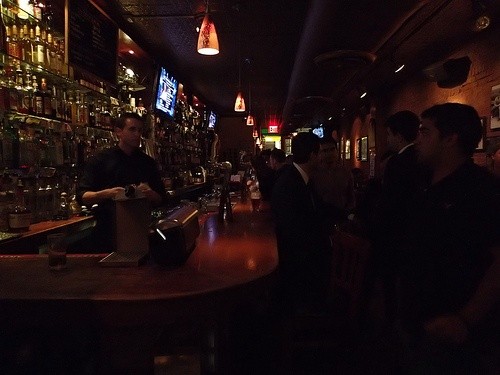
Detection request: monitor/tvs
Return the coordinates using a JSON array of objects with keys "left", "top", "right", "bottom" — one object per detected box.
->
[
  {"left": 151, "top": 64, "right": 178, "bottom": 120},
  {"left": 208, "top": 110, "right": 217, "bottom": 128},
  {"left": 312, "top": 126, "right": 325, "bottom": 139}
]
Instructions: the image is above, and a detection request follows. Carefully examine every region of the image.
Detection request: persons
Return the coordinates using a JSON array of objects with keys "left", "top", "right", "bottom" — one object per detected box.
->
[
  {"left": 251, "top": 101, "right": 500, "bottom": 375},
  {"left": 76, "top": 112, "right": 165, "bottom": 252}
]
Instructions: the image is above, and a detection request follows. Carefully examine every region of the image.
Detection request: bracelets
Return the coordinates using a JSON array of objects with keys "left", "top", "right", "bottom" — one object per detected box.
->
[{"left": 453, "top": 311, "right": 470, "bottom": 331}]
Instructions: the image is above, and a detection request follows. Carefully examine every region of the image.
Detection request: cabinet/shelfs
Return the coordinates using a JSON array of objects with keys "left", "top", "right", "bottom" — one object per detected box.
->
[{"left": 0, "top": 0, "right": 220, "bottom": 228}]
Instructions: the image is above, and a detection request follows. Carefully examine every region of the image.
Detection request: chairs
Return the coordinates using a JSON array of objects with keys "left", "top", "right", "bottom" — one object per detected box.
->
[{"left": 269, "top": 229, "right": 375, "bottom": 374}]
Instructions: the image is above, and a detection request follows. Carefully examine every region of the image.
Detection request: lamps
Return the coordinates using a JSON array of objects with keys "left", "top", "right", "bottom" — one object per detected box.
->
[
  {"left": 196, "top": 0, "right": 263, "bottom": 147},
  {"left": 438, "top": 55, "right": 472, "bottom": 88}
]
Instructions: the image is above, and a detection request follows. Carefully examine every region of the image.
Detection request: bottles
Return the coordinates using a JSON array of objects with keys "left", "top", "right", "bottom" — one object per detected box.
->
[
  {"left": 219, "top": 192, "right": 232, "bottom": 210},
  {"left": 2, "top": 0, "right": 203, "bottom": 233},
  {"left": 210, "top": 164, "right": 229, "bottom": 191}
]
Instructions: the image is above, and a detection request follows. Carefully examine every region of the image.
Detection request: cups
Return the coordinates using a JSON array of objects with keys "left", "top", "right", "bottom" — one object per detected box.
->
[
  {"left": 112, "top": 187, "right": 126, "bottom": 201},
  {"left": 252, "top": 198, "right": 260, "bottom": 213},
  {"left": 48, "top": 235, "right": 68, "bottom": 270}
]
